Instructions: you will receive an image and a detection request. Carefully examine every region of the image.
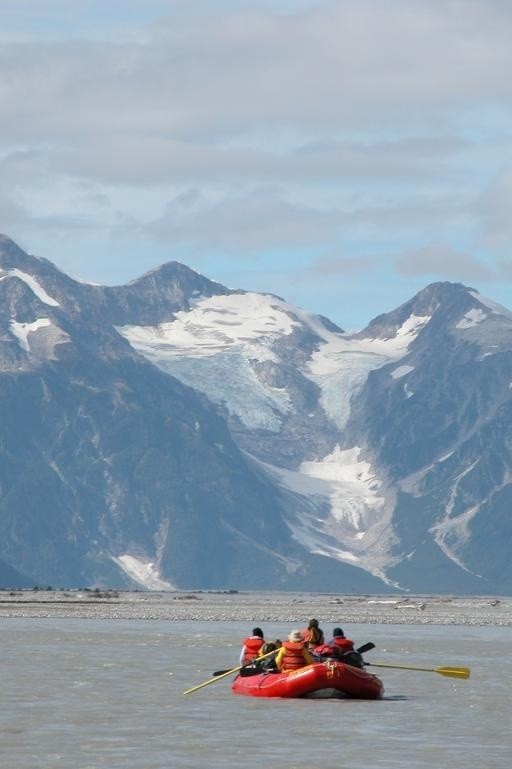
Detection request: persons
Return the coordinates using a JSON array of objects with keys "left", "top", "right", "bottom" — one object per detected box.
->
[
  {"left": 274, "top": 629, "right": 313, "bottom": 674},
  {"left": 251, "top": 642, "right": 278, "bottom": 671},
  {"left": 238, "top": 626, "right": 267, "bottom": 673},
  {"left": 328, "top": 627, "right": 356, "bottom": 653},
  {"left": 305, "top": 618, "right": 324, "bottom": 648}
]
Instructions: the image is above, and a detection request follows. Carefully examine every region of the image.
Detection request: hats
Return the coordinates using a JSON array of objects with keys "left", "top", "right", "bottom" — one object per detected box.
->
[{"left": 288, "top": 630, "right": 305, "bottom": 642}]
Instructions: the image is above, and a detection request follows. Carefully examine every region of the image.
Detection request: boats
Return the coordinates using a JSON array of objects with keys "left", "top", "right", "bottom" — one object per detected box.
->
[{"left": 231, "top": 661, "right": 384, "bottom": 701}]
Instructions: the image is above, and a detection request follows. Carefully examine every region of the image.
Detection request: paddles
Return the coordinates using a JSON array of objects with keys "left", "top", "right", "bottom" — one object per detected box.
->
[
  {"left": 240, "top": 668, "right": 282, "bottom": 676},
  {"left": 356, "top": 642, "right": 375, "bottom": 654},
  {"left": 213, "top": 670, "right": 232, "bottom": 676},
  {"left": 364, "top": 662, "right": 469, "bottom": 679}
]
